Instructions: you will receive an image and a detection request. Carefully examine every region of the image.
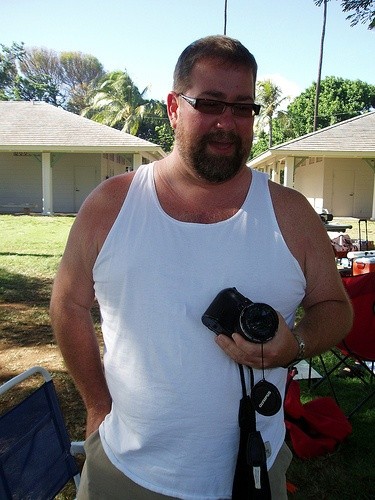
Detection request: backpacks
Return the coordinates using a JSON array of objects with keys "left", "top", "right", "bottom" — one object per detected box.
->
[{"left": 282, "top": 367, "right": 353, "bottom": 461}]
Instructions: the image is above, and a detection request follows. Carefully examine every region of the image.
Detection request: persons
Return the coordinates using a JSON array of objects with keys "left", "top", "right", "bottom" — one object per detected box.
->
[{"left": 50, "top": 36, "right": 352, "bottom": 500}]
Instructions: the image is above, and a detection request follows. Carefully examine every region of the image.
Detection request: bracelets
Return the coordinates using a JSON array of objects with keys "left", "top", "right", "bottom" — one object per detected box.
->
[{"left": 286, "top": 331, "right": 307, "bottom": 370}]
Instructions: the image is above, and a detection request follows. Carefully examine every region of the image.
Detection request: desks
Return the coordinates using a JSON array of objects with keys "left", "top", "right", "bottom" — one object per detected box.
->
[{"left": 324, "top": 224, "right": 352, "bottom": 233}]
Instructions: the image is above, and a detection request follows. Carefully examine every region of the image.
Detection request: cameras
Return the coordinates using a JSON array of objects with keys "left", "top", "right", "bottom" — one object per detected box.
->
[{"left": 201, "top": 287, "right": 279, "bottom": 344}]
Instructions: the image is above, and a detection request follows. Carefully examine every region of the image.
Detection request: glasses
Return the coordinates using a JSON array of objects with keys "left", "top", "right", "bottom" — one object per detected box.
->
[{"left": 175, "top": 92, "right": 262, "bottom": 119}]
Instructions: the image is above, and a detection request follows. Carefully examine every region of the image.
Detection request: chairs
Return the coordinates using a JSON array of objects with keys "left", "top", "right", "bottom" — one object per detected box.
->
[
  {"left": 306, "top": 271, "right": 375, "bottom": 422},
  {"left": 0, "top": 366, "right": 86, "bottom": 500}
]
